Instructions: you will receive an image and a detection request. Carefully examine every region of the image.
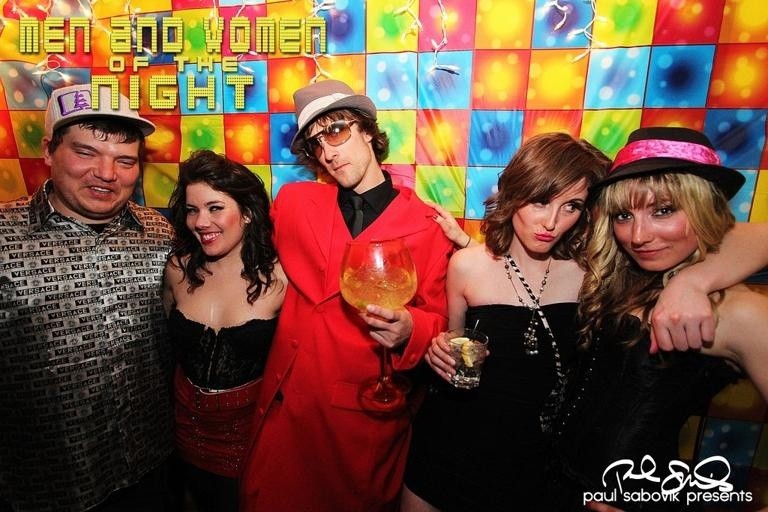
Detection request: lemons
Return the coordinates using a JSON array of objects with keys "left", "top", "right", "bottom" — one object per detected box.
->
[
  {"left": 450, "top": 337, "right": 470, "bottom": 345},
  {"left": 462, "top": 341, "right": 475, "bottom": 367}
]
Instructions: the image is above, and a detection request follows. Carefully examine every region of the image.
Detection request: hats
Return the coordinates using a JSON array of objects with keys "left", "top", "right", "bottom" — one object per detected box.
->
[
  {"left": 586, "top": 126, "right": 747, "bottom": 204},
  {"left": 286, "top": 78, "right": 378, "bottom": 155},
  {"left": 44, "top": 83, "right": 158, "bottom": 144}
]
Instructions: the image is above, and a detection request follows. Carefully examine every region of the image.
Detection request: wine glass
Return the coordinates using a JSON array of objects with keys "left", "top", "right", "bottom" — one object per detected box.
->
[{"left": 339, "top": 239, "right": 419, "bottom": 400}]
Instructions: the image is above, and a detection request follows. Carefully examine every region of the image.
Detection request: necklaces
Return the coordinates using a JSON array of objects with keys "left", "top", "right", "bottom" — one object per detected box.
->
[{"left": 504, "top": 253, "right": 552, "bottom": 355}]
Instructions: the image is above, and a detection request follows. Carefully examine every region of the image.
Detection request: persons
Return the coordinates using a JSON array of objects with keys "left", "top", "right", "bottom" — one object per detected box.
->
[
  {"left": 161, "top": 146, "right": 288, "bottom": 511},
  {"left": 241, "top": 81, "right": 457, "bottom": 512},
  {"left": 1, "top": 82, "right": 175, "bottom": 512},
  {"left": 541, "top": 126, "right": 767, "bottom": 512},
  {"left": 400, "top": 133, "right": 767, "bottom": 512}
]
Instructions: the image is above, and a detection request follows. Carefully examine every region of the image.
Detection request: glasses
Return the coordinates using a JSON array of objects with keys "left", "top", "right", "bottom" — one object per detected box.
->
[{"left": 302, "top": 116, "right": 359, "bottom": 161}]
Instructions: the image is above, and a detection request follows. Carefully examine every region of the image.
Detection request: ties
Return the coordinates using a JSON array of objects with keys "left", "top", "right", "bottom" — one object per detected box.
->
[{"left": 347, "top": 194, "right": 364, "bottom": 243}]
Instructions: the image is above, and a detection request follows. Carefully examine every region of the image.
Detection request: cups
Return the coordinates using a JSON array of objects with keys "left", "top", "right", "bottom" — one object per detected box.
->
[{"left": 444, "top": 329, "right": 489, "bottom": 391}]
